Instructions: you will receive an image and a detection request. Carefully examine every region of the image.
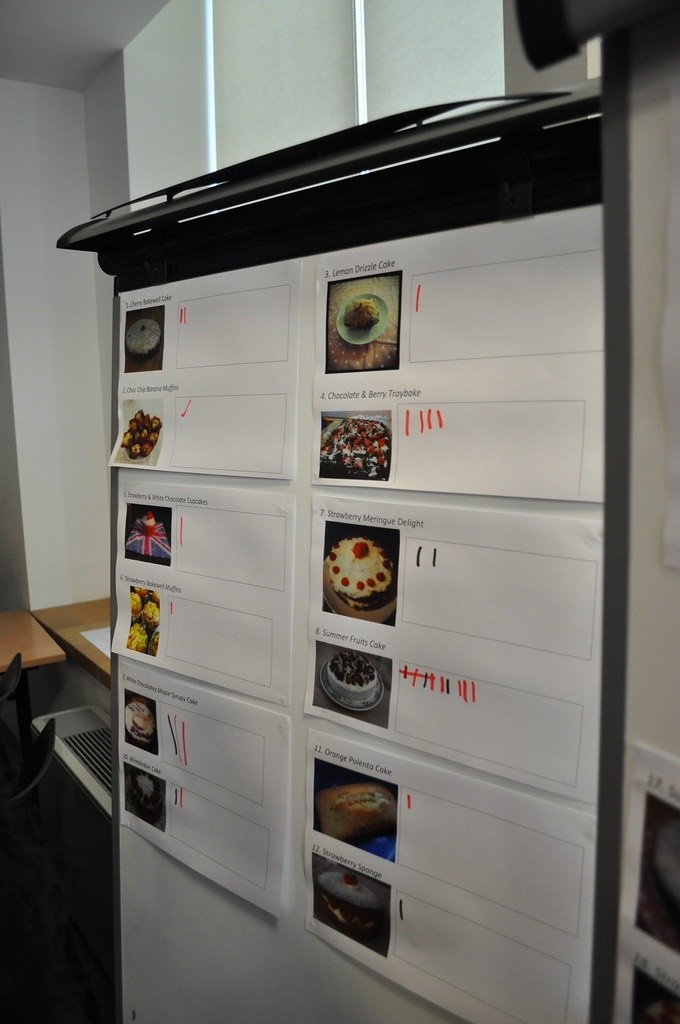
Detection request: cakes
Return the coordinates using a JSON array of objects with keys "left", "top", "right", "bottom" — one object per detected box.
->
[
  {"left": 125, "top": 319, "right": 161, "bottom": 361},
  {"left": 327, "top": 536, "right": 396, "bottom": 610},
  {"left": 315, "top": 870, "right": 380, "bottom": 939},
  {"left": 125, "top": 702, "right": 155, "bottom": 744},
  {"left": 327, "top": 650, "right": 377, "bottom": 703},
  {"left": 319, "top": 418, "right": 390, "bottom": 481},
  {"left": 129, "top": 765, "right": 163, "bottom": 823}
]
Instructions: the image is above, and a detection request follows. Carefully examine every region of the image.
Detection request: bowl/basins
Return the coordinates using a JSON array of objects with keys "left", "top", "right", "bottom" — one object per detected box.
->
[{"left": 317, "top": 880, "right": 384, "bottom": 933}]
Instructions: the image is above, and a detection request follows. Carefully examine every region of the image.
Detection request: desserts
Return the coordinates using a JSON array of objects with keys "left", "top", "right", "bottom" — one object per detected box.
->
[
  {"left": 126, "top": 585, "right": 160, "bottom": 657},
  {"left": 141, "top": 512, "right": 156, "bottom": 537}
]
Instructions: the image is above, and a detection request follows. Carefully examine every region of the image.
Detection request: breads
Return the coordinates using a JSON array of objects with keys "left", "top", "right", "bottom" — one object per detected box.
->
[
  {"left": 312, "top": 783, "right": 397, "bottom": 844},
  {"left": 344, "top": 299, "right": 380, "bottom": 329}
]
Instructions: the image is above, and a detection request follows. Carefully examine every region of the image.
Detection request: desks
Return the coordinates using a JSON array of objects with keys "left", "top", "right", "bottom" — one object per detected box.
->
[
  {"left": 0, "top": 609, "right": 66, "bottom": 846},
  {"left": 29, "top": 598, "right": 111, "bottom": 690}
]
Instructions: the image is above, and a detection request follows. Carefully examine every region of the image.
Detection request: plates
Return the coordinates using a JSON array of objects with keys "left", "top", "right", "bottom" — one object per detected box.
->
[
  {"left": 323, "top": 564, "right": 397, "bottom": 623},
  {"left": 320, "top": 659, "right": 384, "bottom": 711},
  {"left": 336, "top": 294, "right": 388, "bottom": 345},
  {"left": 125, "top": 446, "right": 159, "bottom": 461}
]
